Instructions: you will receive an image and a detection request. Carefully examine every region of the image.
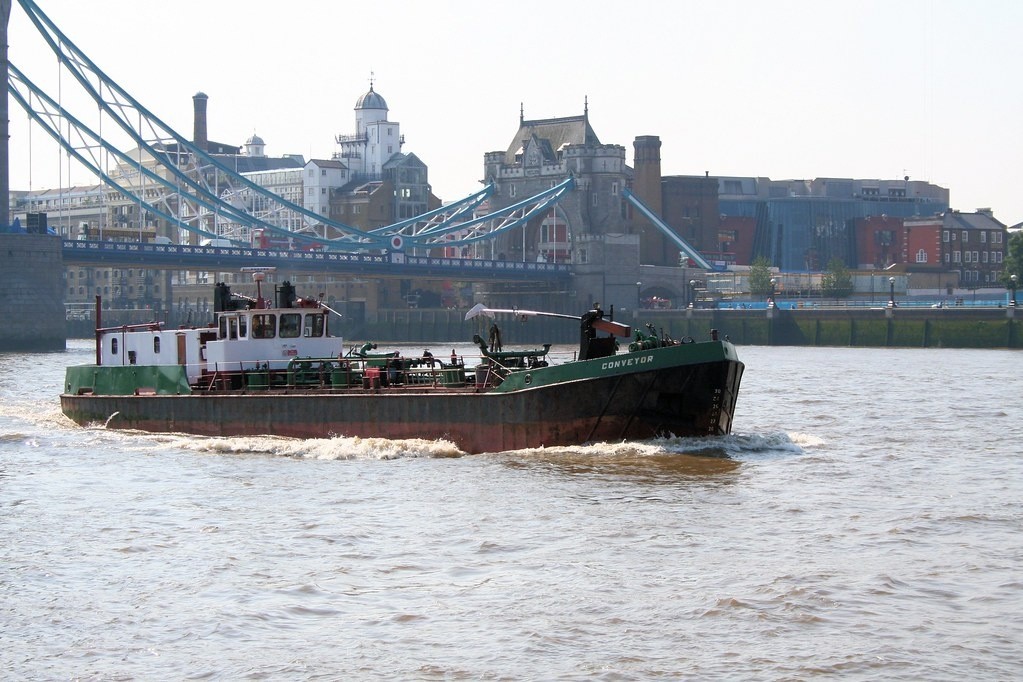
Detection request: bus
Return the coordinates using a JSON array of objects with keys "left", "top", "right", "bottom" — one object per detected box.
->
[
  {"left": 249, "top": 227, "right": 324, "bottom": 249},
  {"left": 676, "top": 249, "right": 738, "bottom": 271}
]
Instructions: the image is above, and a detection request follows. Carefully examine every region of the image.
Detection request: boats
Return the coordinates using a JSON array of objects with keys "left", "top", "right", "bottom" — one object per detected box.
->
[{"left": 59, "top": 261, "right": 747, "bottom": 456}]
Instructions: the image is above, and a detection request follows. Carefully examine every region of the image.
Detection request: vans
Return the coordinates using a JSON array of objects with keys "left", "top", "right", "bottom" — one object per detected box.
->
[
  {"left": 199, "top": 238, "right": 231, "bottom": 247},
  {"left": 154, "top": 236, "right": 174, "bottom": 245}
]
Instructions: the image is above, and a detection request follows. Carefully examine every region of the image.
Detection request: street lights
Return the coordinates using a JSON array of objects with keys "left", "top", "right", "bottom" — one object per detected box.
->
[
  {"left": 1009, "top": 274, "right": 1019, "bottom": 306},
  {"left": 688, "top": 279, "right": 696, "bottom": 307},
  {"left": 768, "top": 278, "right": 777, "bottom": 307},
  {"left": 888, "top": 276, "right": 897, "bottom": 307},
  {"left": 636, "top": 281, "right": 642, "bottom": 308}
]
